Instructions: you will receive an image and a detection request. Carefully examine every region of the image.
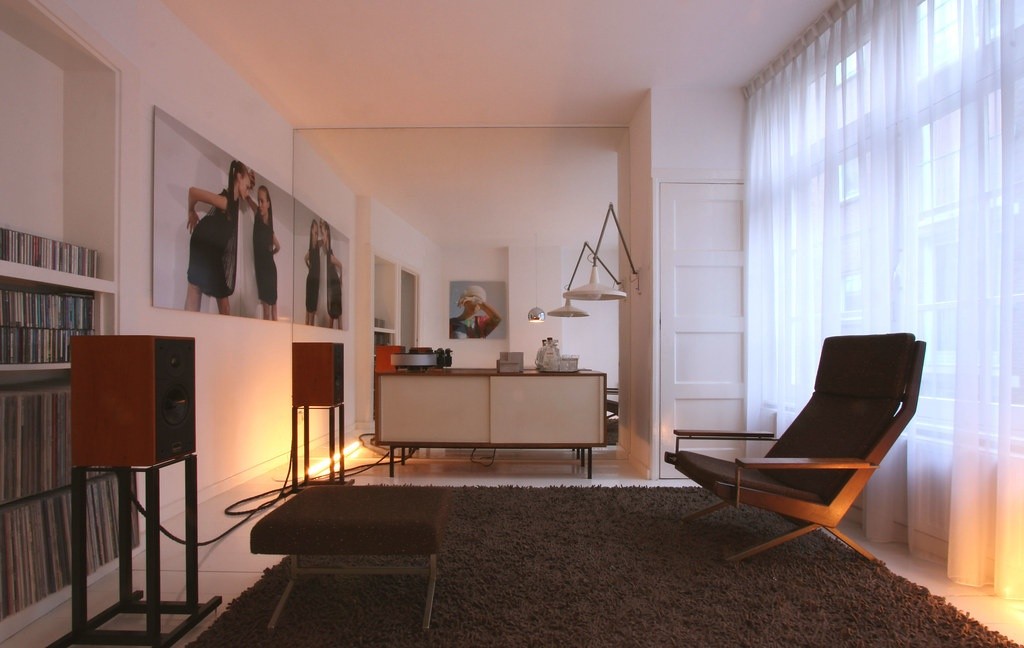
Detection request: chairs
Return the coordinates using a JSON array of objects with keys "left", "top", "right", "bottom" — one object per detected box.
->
[
  {"left": 661, "top": 333, "right": 928, "bottom": 564},
  {"left": 605, "top": 387, "right": 618, "bottom": 418}
]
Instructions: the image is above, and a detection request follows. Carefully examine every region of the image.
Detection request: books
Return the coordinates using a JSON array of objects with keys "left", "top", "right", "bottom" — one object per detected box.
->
[
  {"left": 0, "top": 228, "right": 100, "bottom": 279},
  {"left": 0, "top": 289, "right": 95, "bottom": 364},
  {"left": 0, "top": 380, "right": 141, "bottom": 621}
]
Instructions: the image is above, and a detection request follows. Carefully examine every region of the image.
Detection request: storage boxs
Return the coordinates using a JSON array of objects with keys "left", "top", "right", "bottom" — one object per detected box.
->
[
  {"left": 499, "top": 351, "right": 524, "bottom": 372},
  {"left": 497, "top": 360, "right": 521, "bottom": 373}
]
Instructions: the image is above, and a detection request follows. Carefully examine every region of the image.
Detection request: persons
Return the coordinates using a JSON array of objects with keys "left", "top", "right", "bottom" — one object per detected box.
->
[
  {"left": 246, "top": 168, "right": 280, "bottom": 321},
  {"left": 449, "top": 286, "right": 502, "bottom": 339},
  {"left": 185, "top": 160, "right": 251, "bottom": 315},
  {"left": 304, "top": 218, "right": 343, "bottom": 329}
]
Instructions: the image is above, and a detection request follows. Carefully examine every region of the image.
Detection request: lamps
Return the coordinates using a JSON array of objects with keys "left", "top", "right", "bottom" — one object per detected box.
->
[
  {"left": 563, "top": 201, "right": 643, "bottom": 301},
  {"left": 546, "top": 241, "right": 627, "bottom": 318}
]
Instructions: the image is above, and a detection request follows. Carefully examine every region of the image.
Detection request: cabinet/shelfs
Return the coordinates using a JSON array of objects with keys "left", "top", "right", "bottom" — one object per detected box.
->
[
  {"left": 0, "top": 257, "right": 146, "bottom": 648},
  {"left": 373, "top": 328, "right": 396, "bottom": 357}
]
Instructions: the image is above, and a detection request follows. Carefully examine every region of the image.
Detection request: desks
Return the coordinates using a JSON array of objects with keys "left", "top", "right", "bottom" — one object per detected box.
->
[
  {"left": 248, "top": 484, "right": 454, "bottom": 629},
  {"left": 377, "top": 367, "right": 608, "bottom": 477}
]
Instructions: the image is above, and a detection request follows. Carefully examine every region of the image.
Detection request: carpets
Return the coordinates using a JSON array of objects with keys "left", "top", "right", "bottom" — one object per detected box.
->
[{"left": 189, "top": 485, "right": 1024, "bottom": 648}]
